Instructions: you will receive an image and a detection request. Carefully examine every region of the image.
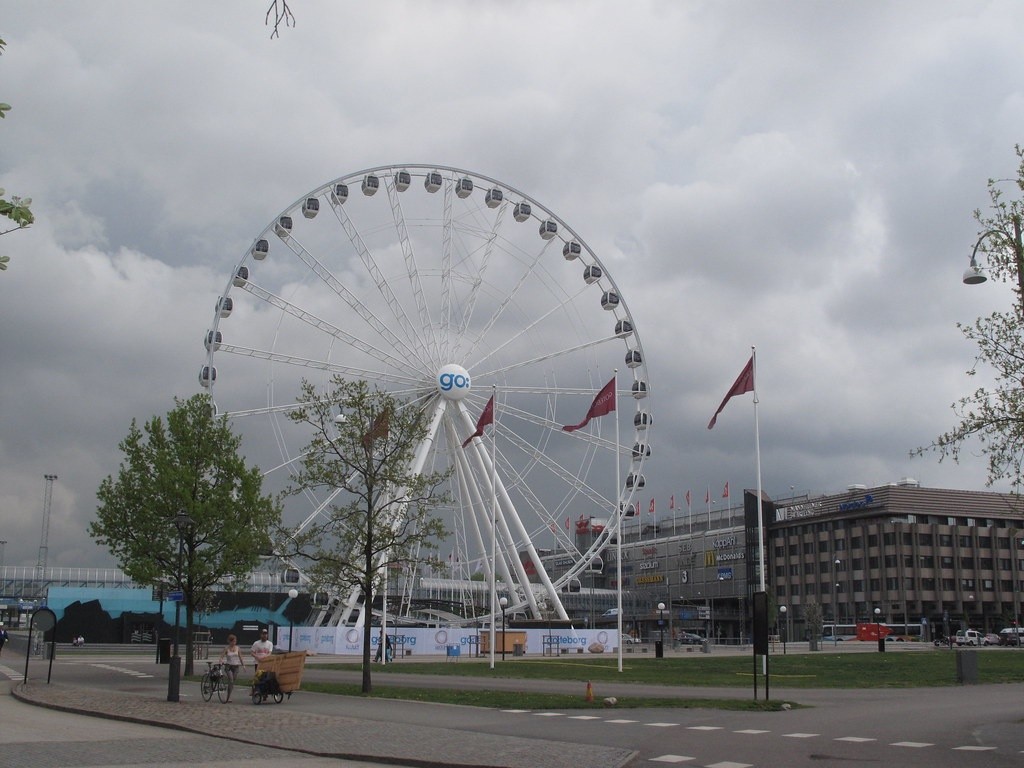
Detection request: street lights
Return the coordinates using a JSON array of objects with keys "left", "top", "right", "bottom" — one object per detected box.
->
[
  {"left": 289, "top": 589, "right": 298, "bottom": 652},
  {"left": 500, "top": 597, "right": 507, "bottom": 661},
  {"left": 780, "top": 606, "right": 786, "bottom": 654},
  {"left": 658, "top": 602, "right": 666, "bottom": 646},
  {"left": 874, "top": 608, "right": 881, "bottom": 652}
]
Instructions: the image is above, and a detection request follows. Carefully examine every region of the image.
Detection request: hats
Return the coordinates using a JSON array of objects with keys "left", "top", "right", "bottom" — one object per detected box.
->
[{"left": 262, "top": 629, "right": 267, "bottom": 633}]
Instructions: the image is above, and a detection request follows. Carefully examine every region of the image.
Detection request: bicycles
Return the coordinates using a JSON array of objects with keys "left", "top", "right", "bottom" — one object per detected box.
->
[{"left": 200, "top": 662, "right": 232, "bottom": 704}]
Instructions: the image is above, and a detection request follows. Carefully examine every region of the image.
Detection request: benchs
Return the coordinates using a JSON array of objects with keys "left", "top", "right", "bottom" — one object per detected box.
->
[
  {"left": 613, "top": 646, "right": 648, "bottom": 653},
  {"left": 546, "top": 647, "right": 583, "bottom": 654},
  {"left": 674, "top": 646, "right": 702, "bottom": 652}
]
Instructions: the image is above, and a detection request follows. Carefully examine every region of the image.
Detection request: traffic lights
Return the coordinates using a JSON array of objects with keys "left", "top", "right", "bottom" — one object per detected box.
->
[{"left": 1011, "top": 620, "right": 1016, "bottom": 628}]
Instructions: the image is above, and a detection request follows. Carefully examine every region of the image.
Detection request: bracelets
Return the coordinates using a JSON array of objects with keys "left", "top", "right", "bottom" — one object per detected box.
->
[{"left": 243, "top": 666, "right": 245, "bottom": 669}]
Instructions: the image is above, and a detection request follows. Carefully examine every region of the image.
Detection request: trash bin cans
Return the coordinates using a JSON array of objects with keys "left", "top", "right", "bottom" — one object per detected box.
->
[
  {"left": 45, "top": 642, "right": 56, "bottom": 661},
  {"left": 879, "top": 638, "right": 885, "bottom": 653},
  {"left": 513, "top": 644, "right": 523, "bottom": 657},
  {"left": 654, "top": 640, "right": 662, "bottom": 658},
  {"left": 160, "top": 638, "right": 174, "bottom": 664},
  {"left": 447, "top": 643, "right": 460, "bottom": 656}
]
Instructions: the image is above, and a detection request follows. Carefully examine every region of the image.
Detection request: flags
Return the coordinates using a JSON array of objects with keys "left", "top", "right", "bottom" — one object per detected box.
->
[
  {"left": 706, "top": 351, "right": 756, "bottom": 428},
  {"left": 560, "top": 378, "right": 617, "bottom": 432},
  {"left": 462, "top": 393, "right": 493, "bottom": 449}
]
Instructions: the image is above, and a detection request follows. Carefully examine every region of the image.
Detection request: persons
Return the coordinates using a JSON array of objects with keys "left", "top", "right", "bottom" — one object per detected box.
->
[
  {"left": 73, "top": 635, "right": 84, "bottom": 647},
  {"left": 373, "top": 631, "right": 393, "bottom": 662},
  {"left": 0, "top": 624, "right": 8, "bottom": 651},
  {"left": 219, "top": 635, "right": 246, "bottom": 702},
  {"left": 250, "top": 629, "right": 273, "bottom": 702}
]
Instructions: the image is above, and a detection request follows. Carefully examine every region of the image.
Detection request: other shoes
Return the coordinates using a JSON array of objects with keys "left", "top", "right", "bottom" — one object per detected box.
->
[{"left": 373, "top": 660, "right": 377, "bottom": 662}]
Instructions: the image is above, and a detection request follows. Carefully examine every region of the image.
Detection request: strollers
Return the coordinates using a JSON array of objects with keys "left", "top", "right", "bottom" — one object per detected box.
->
[{"left": 250, "top": 667, "right": 285, "bottom": 704}]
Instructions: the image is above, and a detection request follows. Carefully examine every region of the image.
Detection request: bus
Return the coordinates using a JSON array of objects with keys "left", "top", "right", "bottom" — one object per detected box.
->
[{"left": 817, "top": 623, "right": 925, "bottom": 643}]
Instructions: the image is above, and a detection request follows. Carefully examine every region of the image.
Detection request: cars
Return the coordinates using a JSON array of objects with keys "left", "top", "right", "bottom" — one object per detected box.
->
[
  {"left": 955, "top": 627, "right": 1024, "bottom": 647},
  {"left": 621, "top": 633, "right": 641, "bottom": 644},
  {"left": 677, "top": 632, "right": 707, "bottom": 646}
]
